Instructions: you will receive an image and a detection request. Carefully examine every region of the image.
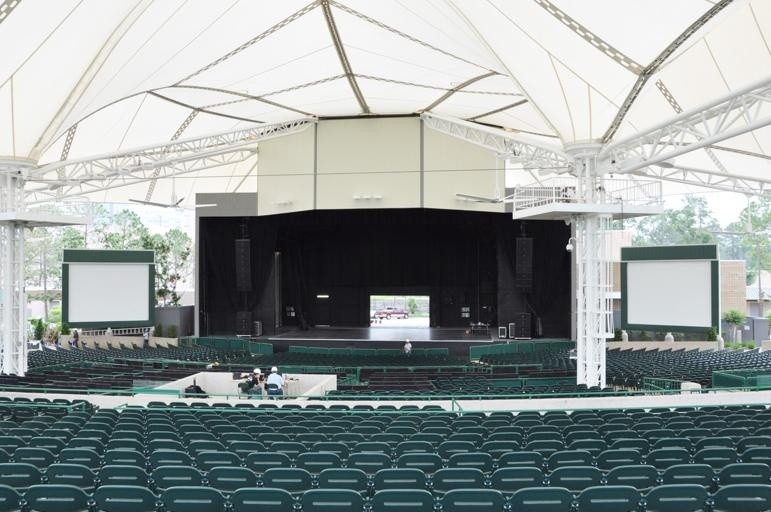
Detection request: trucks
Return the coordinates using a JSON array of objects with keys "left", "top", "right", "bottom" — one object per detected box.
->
[{"left": 375, "top": 306, "right": 408, "bottom": 320}]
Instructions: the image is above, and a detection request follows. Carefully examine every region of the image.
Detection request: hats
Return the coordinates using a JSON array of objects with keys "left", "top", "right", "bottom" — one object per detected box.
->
[
  {"left": 271, "top": 367, "right": 277, "bottom": 372},
  {"left": 254, "top": 368, "right": 264, "bottom": 375}
]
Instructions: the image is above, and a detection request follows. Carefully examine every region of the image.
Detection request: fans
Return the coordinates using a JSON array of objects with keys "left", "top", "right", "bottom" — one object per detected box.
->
[
  {"left": 127, "top": 174, "right": 216, "bottom": 210},
  {"left": 454, "top": 153, "right": 538, "bottom": 205}
]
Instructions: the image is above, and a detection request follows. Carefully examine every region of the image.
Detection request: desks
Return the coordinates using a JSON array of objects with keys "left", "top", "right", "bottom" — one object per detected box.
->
[
  {"left": 470, "top": 324, "right": 490, "bottom": 336},
  {"left": 230, "top": 374, "right": 303, "bottom": 399}
]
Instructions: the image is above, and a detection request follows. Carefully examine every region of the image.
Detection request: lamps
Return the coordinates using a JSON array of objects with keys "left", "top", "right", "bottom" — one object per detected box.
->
[{"left": 565, "top": 237, "right": 574, "bottom": 252}]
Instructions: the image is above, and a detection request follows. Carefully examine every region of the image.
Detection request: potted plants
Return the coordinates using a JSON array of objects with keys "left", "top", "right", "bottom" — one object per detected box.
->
[{"left": 722, "top": 307, "right": 747, "bottom": 343}]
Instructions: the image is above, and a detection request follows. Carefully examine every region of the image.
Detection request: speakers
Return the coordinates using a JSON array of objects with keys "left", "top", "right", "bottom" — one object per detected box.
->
[
  {"left": 236, "top": 312, "right": 253, "bottom": 335},
  {"left": 515, "top": 237, "right": 534, "bottom": 296},
  {"left": 516, "top": 312, "right": 534, "bottom": 337},
  {"left": 235, "top": 239, "right": 253, "bottom": 291}
]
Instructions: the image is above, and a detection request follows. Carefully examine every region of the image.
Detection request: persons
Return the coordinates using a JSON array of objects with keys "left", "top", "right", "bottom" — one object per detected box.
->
[
  {"left": 241, "top": 368, "right": 262, "bottom": 399},
  {"left": 143, "top": 329, "right": 149, "bottom": 345},
  {"left": 74, "top": 330, "right": 78, "bottom": 348},
  {"left": 404, "top": 340, "right": 412, "bottom": 355},
  {"left": 266, "top": 367, "right": 284, "bottom": 400}
]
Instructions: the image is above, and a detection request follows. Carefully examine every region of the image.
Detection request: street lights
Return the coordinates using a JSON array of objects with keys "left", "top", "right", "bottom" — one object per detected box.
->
[{"left": 566, "top": 235, "right": 586, "bottom": 386}]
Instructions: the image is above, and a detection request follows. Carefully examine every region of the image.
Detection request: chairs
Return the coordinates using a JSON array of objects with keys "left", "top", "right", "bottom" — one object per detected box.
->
[
  {"left": 2, "top": 342, "right": 770, "bottom": 395},
  {"left": 0, "top": 397, "right": 771, "bottom": 512}
]
